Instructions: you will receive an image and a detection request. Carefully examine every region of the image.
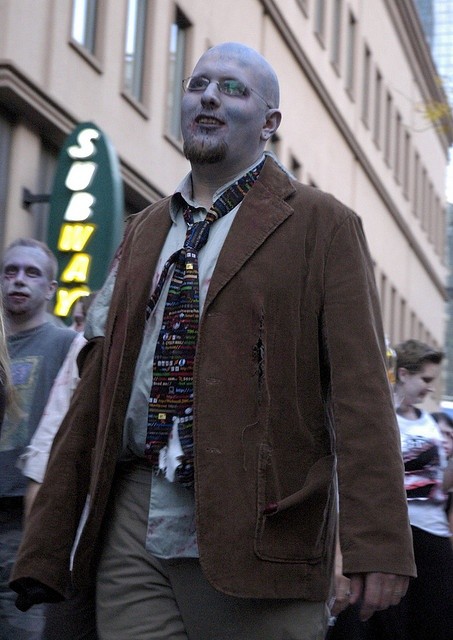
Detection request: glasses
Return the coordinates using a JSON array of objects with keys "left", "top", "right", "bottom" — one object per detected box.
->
[{"left": 182, "top": 77, "right": 278, "bottom": 108}]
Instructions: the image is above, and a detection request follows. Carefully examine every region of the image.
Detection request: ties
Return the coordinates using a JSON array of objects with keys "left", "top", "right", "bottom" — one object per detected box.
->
[{"left": 146, "top": 163, "right": 262, "bottom": 489}]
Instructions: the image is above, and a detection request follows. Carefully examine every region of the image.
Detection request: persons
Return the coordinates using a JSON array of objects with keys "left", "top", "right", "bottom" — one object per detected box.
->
[
  {"left": 0, "top": 238, "right": 87, "bottom": 640},
  {"left": 363, "top": 340, "right": 452, "bottom": 639},
  {"left": 64, "top": 293, "right": 98, "bottom": 337},
  {"left": 430, "top": 412, "right": 453, "bottom": 490},
  {"left": 6, "top": 40, "right": 419, "bottom": 640}
]
